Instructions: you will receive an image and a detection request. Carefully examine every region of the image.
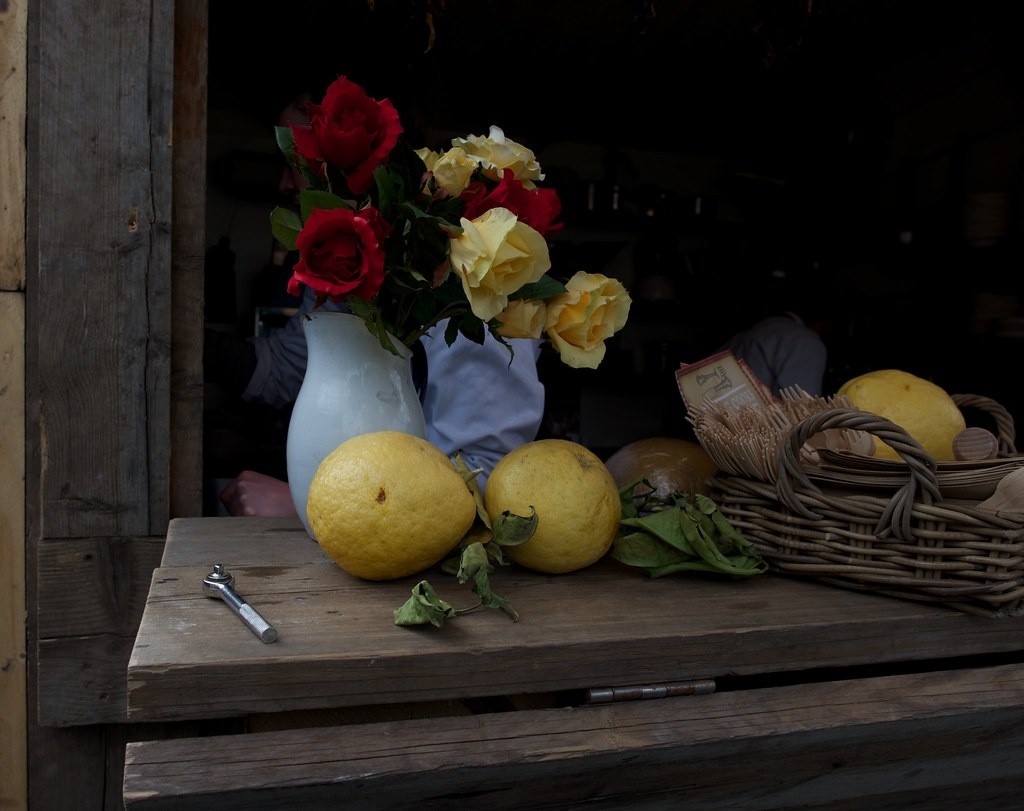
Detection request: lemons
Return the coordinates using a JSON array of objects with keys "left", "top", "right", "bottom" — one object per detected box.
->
[
  {"left": 483, "top": 439, "right": 622, "bottom": 573},
  {"left": 834, "top": 369, "right": 965, "bottom": 461},
  {"left": 307, "top": 432, "right": 476, "bottom": 582}
]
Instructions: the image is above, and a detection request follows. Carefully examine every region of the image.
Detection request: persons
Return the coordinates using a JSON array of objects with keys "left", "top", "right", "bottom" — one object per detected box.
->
[
  {"left": 242, "top": 244, "right": 308, "bottom": 411},
  {"left": 411, "top": 316, "right": 546, "bottom": 498},
  {"left": 719, "top": 302, "right": 828, "bottom": 396}
]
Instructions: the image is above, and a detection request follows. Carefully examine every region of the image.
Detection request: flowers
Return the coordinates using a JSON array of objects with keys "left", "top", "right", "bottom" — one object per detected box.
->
[{"left": 265, "top": 75, "right": 632, "bottom": 373}]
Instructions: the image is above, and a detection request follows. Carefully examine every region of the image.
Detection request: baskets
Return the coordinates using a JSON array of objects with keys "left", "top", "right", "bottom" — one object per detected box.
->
[{"left": 708, "top": 394, "right": 1024, "bottom": 619}]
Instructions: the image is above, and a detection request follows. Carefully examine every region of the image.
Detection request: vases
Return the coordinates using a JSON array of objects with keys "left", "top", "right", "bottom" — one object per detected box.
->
[{"left": 285, "top": 309, "right": 426, "bottom": 541}]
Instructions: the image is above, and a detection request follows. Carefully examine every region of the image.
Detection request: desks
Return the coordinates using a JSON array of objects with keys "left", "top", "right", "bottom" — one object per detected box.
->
[{"left": 122, "top": 517, "right": 1024, "bottom": 811}]
